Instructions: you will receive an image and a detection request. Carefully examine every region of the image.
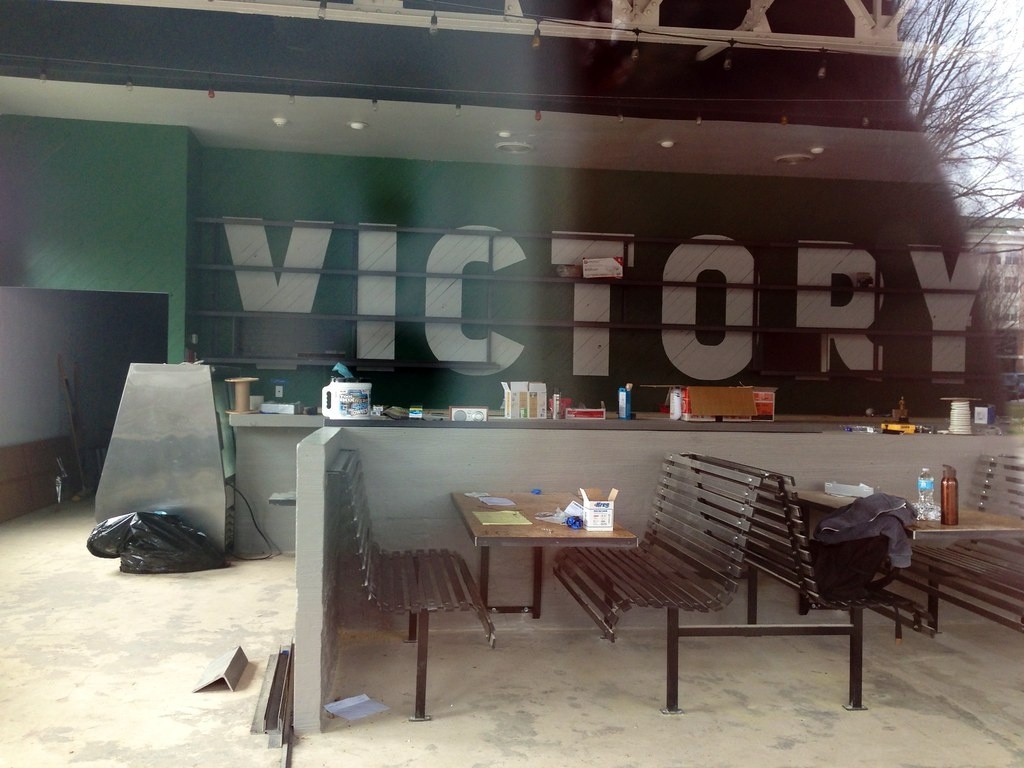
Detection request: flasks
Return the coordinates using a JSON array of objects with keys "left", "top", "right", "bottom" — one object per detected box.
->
[
  {"left": 552, "top": 386, "right": 562, "bottom": 420},
  {"left": 941, "top": 464, "right": 958, "bottom": 525}
]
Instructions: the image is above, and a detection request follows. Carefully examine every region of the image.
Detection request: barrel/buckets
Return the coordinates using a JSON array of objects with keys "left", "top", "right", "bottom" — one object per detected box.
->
[{"left": 321, "top": 376, "right": 372, "bottom": 420}]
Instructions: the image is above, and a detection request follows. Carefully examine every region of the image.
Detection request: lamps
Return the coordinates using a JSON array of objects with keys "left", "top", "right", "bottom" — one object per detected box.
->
[{"left": 37, "top": 0, "right": 869, "bottom": 127}]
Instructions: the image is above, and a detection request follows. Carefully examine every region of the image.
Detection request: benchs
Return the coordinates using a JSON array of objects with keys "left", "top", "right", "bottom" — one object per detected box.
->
[{"left": 327, "top": 448, "right": 1024, "bottom": 721}]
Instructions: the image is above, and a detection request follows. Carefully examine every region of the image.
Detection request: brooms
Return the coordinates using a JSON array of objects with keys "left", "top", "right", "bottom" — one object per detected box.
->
[{"left": 56, "top": 357, "right": 96, "bottom": 503}]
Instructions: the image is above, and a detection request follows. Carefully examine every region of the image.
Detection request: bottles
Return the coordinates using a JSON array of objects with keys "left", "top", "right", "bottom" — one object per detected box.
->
[
  {"left": 669, "top": 386, "right": 682, "bottom": 419},
  {"left": 917, "top": 468, "right": 935, "bottom": 521}
]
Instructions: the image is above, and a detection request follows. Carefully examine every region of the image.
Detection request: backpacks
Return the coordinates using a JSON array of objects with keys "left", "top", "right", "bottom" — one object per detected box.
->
[{"left": 808, "top": 533, "right": 889, "bottom": 607}]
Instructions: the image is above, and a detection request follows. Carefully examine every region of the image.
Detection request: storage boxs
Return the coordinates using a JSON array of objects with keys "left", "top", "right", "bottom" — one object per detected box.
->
[
  {"left": 565, "top": 400, "right": 606, "bottom": 421},
  {"left": 501, "top": 381, "right": 547, "bottom": 420},
  {"left": 640, "top": 384, "right": 758, "bottom": 422},
  {"left": 751, "top": 387, "right": 778, "bottom": 422}
]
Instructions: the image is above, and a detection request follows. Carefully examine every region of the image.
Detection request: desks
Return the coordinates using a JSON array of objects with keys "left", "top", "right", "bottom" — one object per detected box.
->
[
  {"left": 799, "top": 488, "right": 1024, "bottom": 542},
  {"left": 451, "top": 491, "right": 637, "bottom": 620}
]
